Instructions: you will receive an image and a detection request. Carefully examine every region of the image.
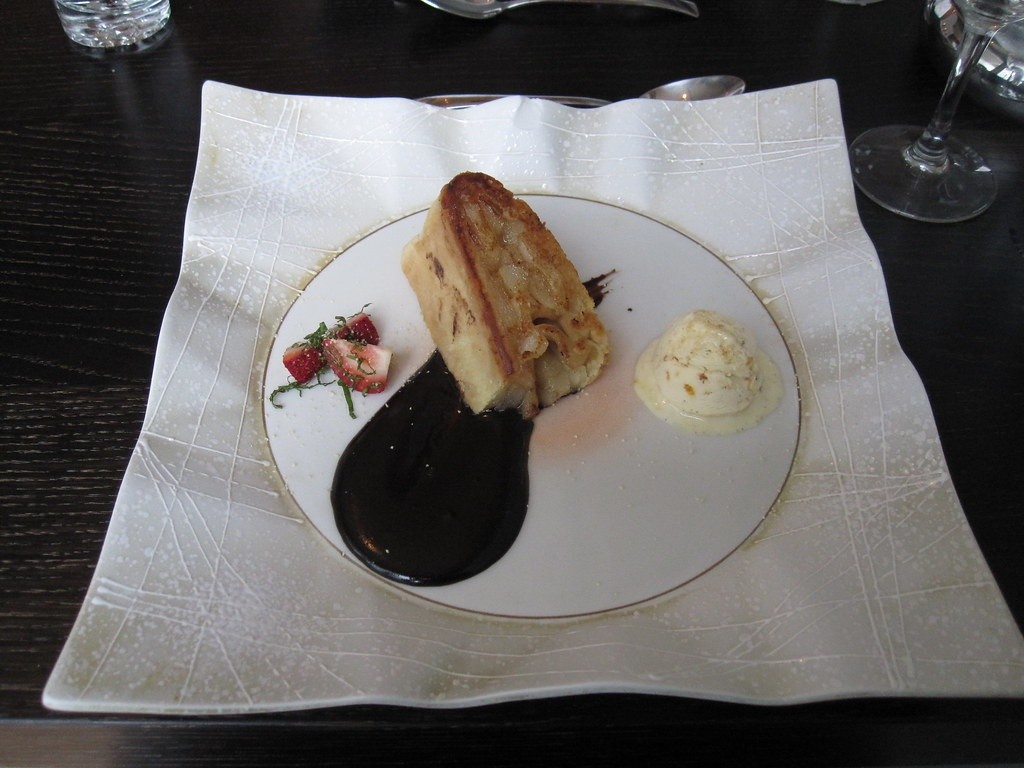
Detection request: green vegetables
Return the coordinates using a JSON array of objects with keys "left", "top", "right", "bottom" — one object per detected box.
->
[{"left": 268, "top": 302, "right": 383, "bottom": 419}]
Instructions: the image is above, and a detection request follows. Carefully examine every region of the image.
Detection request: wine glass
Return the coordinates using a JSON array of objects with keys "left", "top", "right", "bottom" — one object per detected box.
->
[{"left": 846, "top": 0, "right": 1024, "bottom": 224}]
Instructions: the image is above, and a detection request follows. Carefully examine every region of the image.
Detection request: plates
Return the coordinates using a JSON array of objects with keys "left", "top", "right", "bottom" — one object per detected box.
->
[{"left": 38, "top": 79, "right": 1024, "bottom": 716}]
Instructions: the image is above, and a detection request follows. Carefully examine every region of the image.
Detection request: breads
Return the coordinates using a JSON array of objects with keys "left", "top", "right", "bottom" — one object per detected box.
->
[{"left": 401, "top": 172, "right": 612, "bottom": 415}]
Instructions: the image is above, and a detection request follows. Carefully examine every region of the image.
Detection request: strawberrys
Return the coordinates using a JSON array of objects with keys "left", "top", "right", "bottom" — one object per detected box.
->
[{"left": 282, "top": 312, "right": 392, "bottom": 395}]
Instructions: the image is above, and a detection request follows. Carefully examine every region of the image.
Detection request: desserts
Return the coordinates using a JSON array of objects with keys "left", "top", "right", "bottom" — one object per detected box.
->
[{"left": 634, "top": 310, "right": 781, "bottom": 433}]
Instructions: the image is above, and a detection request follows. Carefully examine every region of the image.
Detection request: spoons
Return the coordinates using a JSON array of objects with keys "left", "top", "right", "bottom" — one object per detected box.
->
[
  {"left": 415, "top": 73, "right": 746, "bottom": 107},
  {"left": 421, "top": 0, "right": 700, "bottom": 19}
]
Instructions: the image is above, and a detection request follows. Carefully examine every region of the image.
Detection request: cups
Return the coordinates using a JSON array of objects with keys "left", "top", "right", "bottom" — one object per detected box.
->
[
  {"left": 53, "top": 0, "right": 171, "bottom": 48},
  {"left": 924, "top": 0, "right": 1024, "bottom": 88}
]
